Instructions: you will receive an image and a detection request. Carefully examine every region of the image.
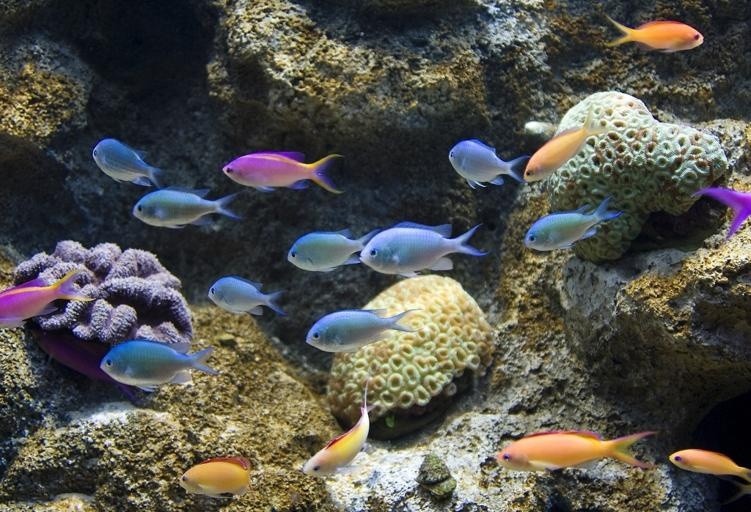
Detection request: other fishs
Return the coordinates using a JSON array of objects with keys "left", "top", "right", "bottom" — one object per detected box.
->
[{"left": 603, "top": 12, "right": 705, "bottom": 55}]
